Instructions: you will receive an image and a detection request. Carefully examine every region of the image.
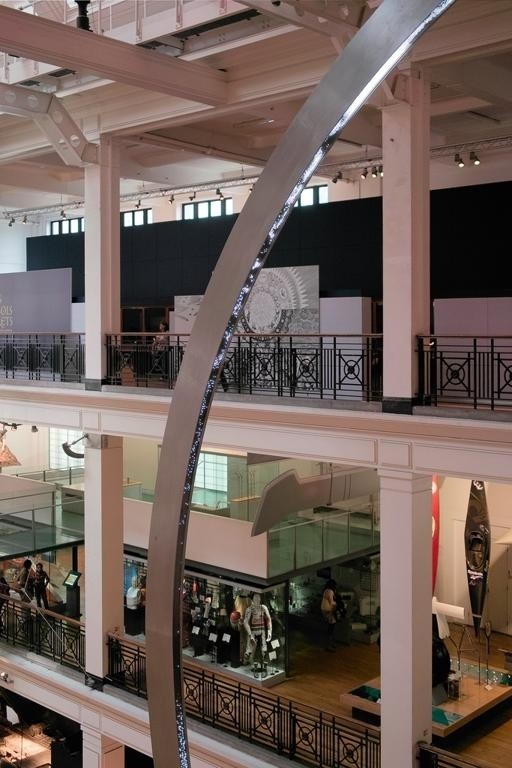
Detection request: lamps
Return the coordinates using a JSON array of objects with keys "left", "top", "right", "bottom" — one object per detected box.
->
[{"left": 2, "top": 131, "right": 511, "bottom": 227}]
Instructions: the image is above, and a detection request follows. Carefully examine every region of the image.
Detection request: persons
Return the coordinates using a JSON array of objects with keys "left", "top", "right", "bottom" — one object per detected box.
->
[
  {"left": 127, "top": 576, "right": 142, "bottom": 609},
  {"left": 321, "top": 578, "right": 337, "bottom": 653},
  {"left": 156, "top": 319, "right": 169, "bottom": 381},
  {"left": 34, "top": 562, "right": 50, "bottom": 610},
  {"left": 244, "top": 594, "right": 272, "bottom": 670},
  {"left": 20, "top": 559, "right": 34, "bottom": 621},
  {"left": 0, "top": 569, "right": 10, "bottom": 627}
]
